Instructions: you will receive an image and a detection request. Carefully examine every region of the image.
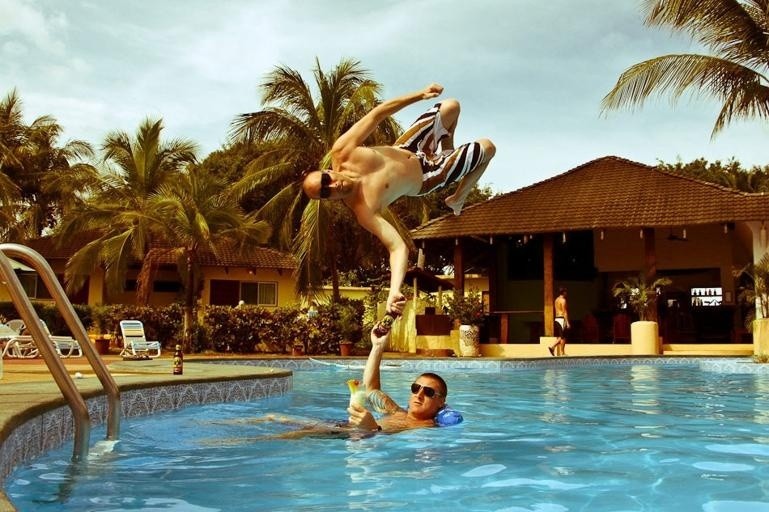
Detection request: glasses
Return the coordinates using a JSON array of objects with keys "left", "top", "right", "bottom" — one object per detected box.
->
[
  {"left": 320, "top": 172, "right": 331, "bottom": 198},
  {"left": 411, "top": 383, "right": 442, "bottom": 399}
]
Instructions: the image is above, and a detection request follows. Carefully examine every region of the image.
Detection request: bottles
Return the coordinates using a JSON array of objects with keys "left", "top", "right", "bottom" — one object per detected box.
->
[
  {"left": 172, "top": 344, "right": 183, "bottom": 375},
  {"left": 372, "top": 295, "right": 405, "bottom": 338}
]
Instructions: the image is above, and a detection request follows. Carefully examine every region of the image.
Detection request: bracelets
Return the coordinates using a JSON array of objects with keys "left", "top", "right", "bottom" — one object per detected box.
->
[{"left": 372, "top": 426, "right": 383, "bottom": 434}]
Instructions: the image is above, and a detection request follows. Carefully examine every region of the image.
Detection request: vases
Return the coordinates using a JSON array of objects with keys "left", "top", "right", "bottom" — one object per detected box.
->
[{"left": 459, "top": 324, "right": 481, "bottom": 358}]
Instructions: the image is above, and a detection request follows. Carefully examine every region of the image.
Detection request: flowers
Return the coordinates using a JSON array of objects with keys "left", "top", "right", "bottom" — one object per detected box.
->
[{"left": 442, "top": 284, "right": 490, "bottom": 328}]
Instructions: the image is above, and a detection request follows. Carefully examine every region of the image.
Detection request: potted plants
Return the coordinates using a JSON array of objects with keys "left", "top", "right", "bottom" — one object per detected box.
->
[
  {"left": 730, "top": 249, "right": 769, "bottom": 356},
  {"left": 333, "top": 301, "right": 360, "bottom": 356},
  {"left": 610, "top": 273, "right": 673, "bottom": 356}
]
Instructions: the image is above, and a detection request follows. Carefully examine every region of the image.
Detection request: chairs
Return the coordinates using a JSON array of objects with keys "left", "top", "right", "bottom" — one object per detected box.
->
[{"left": 0, "top": 315, "right": 163, "bottom": 361}]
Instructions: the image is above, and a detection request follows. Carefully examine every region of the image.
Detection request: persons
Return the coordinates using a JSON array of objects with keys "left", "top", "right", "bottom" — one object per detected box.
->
[
  {"left": 302, "top": 83, "right": 497, "bottom": 319},
  {"left": 185, "top": 319, "right": 450, "bottom": 448},
  {"left": 548, "top": 286, "right": 571, "bottom": 356}
]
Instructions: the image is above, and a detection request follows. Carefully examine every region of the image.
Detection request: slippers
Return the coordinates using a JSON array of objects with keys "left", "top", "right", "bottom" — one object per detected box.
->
[{"left": 548, "top": 347, "right": 555, "bottom": 356}]
